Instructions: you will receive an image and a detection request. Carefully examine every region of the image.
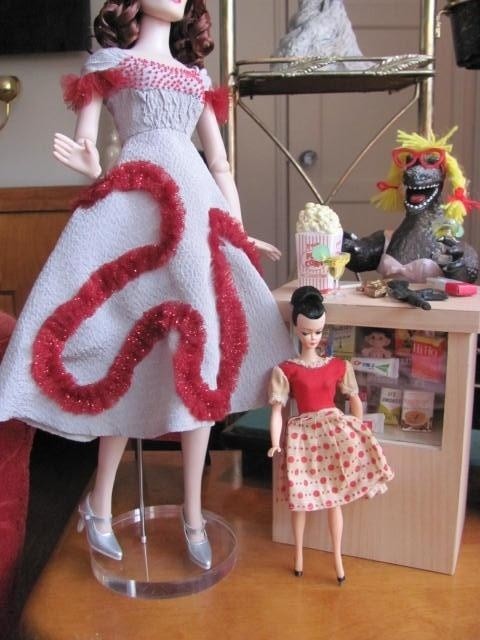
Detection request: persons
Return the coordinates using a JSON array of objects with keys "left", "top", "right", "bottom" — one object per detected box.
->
[
  {"left": 267, "top": 288, "right": 397, "bottom": 584},
  {"left": 0, "top": 1, "right": 281, "bottom": 571},
  {"left": 359, "top": 328, "right": 393, "bottom": 359}
]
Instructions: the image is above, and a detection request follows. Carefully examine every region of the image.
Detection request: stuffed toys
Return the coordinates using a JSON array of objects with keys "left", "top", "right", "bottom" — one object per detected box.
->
[{"left": 343, "top": 127, "right": 479, "bottom": 284}]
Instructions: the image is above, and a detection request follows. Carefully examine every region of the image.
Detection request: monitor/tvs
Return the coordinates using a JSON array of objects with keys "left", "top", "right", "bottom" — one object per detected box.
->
[{"left": 0, "top": 0, "right": 92, "bottom": 55}]
